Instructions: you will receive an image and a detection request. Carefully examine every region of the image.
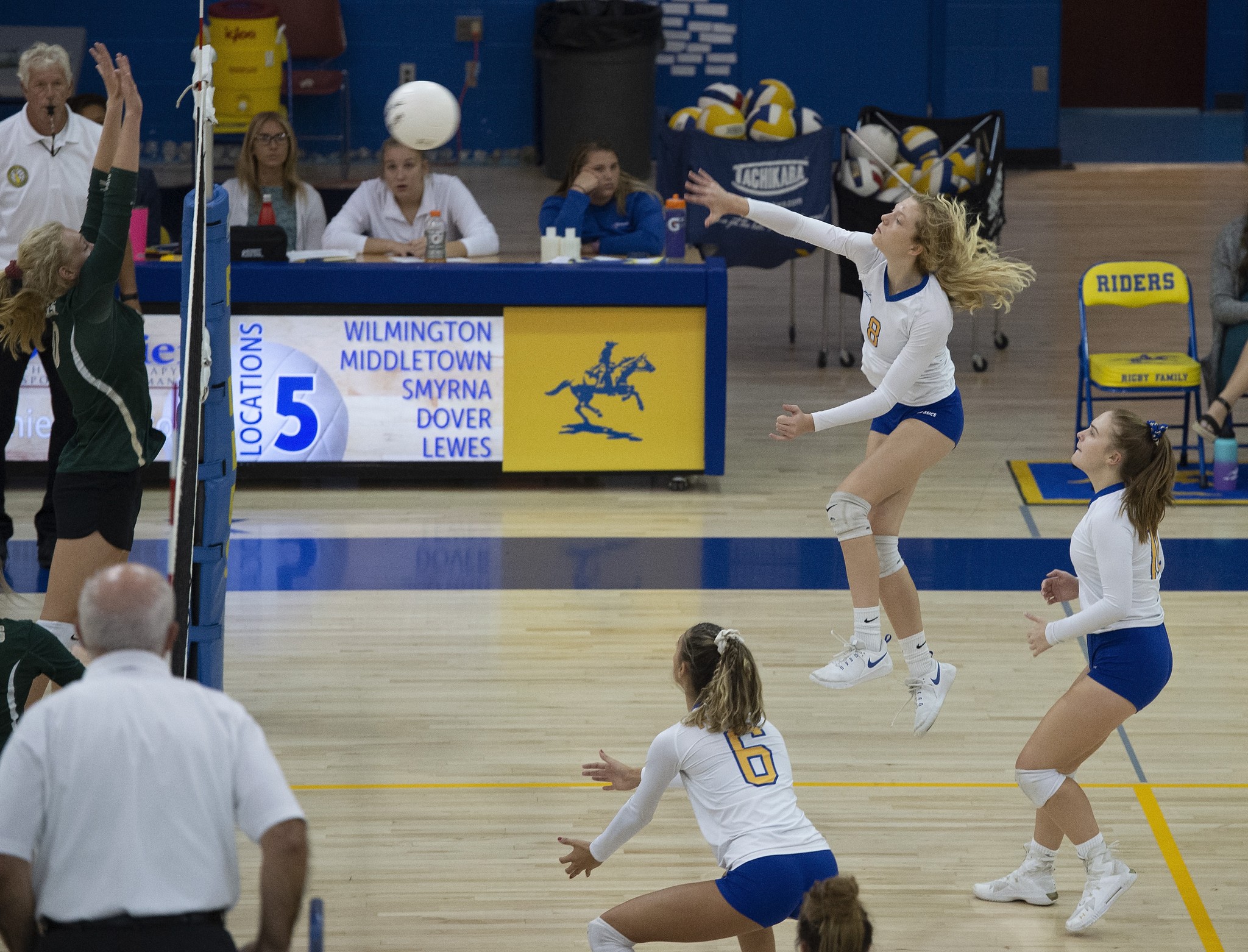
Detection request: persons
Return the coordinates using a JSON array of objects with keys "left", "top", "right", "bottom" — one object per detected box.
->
[{"left": 0, "top": 43, "right": 1248, "bottom": 952}]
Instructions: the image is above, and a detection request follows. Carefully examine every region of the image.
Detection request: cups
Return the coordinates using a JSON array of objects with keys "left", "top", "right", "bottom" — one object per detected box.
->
[{"left": 129, "top": 205, "right": 148, "bottom": 261}]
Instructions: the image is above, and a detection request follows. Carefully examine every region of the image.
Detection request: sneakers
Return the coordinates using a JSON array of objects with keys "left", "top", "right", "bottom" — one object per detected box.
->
[
  {"left": 890, "top": 651, "right": 957, "bottom": 732},
  {"left": 1065, "top": 839, "right": 1138, "bottom": 933},
  {"left": 971, "top": 841, "right": 1058, "bottom": 906},
  {"left": 808, "top": 630, "right": 893, "bottom": 689}
]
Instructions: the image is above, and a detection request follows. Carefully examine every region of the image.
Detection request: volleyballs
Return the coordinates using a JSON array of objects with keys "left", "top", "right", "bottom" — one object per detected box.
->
[
  {"left": 665, "top": 76, "right": 825, "bottom": 142},
  {"left": 836, "top": 124, "right": 982, "bottom": 206},
  {"left": 383, "top": 81, "right": 461, "bottom": 151}
]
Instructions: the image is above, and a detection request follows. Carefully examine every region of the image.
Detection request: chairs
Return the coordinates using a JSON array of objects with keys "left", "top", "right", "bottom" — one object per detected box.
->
[
  {"left": 1074, "top": 259, "right": 1208, "bottom": 489},
  {"left": 146, "top": 185, "right": 194, "bottom": 260},
  {"left": 194, "top": 0, "right": 351, "bottom": 183}
]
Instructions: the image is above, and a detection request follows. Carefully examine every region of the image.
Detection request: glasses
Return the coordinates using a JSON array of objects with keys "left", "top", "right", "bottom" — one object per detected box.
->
[{"left": 254, "top": 131, "right": 289, "bottom": 145}]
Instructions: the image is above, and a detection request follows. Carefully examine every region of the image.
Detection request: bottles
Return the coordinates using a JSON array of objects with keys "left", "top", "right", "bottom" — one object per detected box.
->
[
  {"left": 540, "top": 227, "right": 562, "bottom": 263},
  {"left": 1213, "top": 428, "right": 1239, "bottom": 491},
  {"left": 258, "top": 194, "right": 276, "bottom": 226},
  {"left": 424, "top": 211, "right": 447, "bottom": 264},
  {"left": 560, "top": 227, "right": 582, "bottom": 263},
  {"left": 664, "top": 193, "right": 688, "bottom": 258}
]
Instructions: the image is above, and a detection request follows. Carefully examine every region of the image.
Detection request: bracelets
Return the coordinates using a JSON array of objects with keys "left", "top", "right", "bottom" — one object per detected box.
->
[
  {"left": 572, "top": 184, "right": 587, "bottom": 194},
  {"left": 119, "top": 291, "right": 139, "bottom": 303}
]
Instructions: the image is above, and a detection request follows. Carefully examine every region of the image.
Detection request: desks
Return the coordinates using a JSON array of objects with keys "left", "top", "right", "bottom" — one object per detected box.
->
[{"left": 134, "top": 254, "right": 727, "bottom": 493}]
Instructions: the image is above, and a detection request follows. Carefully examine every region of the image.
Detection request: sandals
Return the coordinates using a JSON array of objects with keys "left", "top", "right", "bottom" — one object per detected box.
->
[{"left": 1191, "top": 397, "right": 1233, "bottom": 443}]
